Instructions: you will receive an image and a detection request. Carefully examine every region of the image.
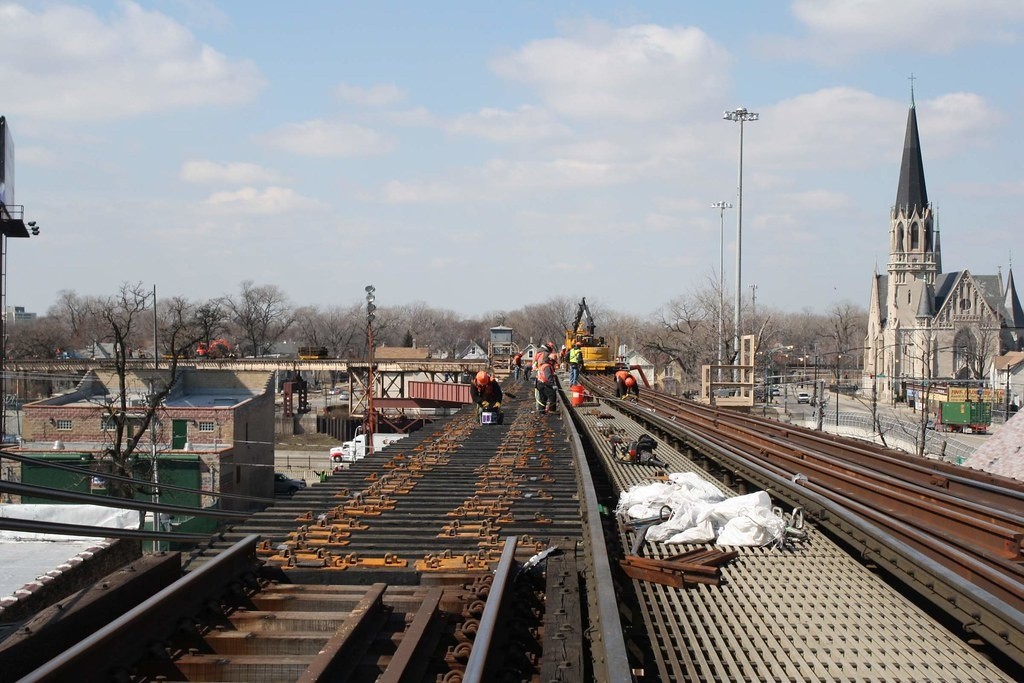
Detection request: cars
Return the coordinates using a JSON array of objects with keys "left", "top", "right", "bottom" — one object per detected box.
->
[
  {"left": 773, "top": 387, "right": 781, "bottom": 397},
  {"left": 797, "top": 392, "right": 811, "bottom": 404}
]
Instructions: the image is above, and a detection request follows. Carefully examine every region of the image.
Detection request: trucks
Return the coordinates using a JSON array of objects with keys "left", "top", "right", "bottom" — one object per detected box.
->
[{"left": 330, "top": 432, "right": 410, "bottom": 462}]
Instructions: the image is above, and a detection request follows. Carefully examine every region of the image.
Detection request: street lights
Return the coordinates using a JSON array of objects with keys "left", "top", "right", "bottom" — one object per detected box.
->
[
  {"left": 836, "top": 347, "right": 872, "bottom": 425},
  {"left": 747, "top": 283, "right": 758, "bottom": 320},
  {"left": 724, "top": 107, "right": 760, "bottom": 400},
  {"left": 872, "top": 339, "right": 914, "bottom": 433},
  {"left": 921, "top": 344, "right": 968, "bottom": 428},
  {"left": 713, "top": 199, "right": 735, "bottom": 395}
]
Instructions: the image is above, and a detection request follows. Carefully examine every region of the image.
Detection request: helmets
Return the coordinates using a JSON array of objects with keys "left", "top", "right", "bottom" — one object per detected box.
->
[
  {"left": 548, "top": 354, "right": 557, "bottom": 364},
  {"left": 547, "top": 342, "right": 555, "bottom": 351},
  {"left": 562, "top": 347, "right": 565, "bottom": 349},
  {"left": 519, "top": 351, "right": 523, "bottom": 355},
  {"left": 624, "top": 377, "right": 634, "bottom": 388},
  {"left": 476, "top": 371, "right": 489, "bottom": 385},
  {"left": 576, "top": 341, "right": 582, "bottom": 346}
]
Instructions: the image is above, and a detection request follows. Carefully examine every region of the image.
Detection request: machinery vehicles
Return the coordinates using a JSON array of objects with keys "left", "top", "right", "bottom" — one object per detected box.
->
[{"left": 563, "top": 296, "right": 616, "bottom": 374}]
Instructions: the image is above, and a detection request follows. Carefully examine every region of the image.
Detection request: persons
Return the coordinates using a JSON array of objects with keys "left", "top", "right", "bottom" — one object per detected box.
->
[
  {"left": 523, "top": 341, "right": 567, "bottom": 417},
  {"left": 614, "top": 370, "right": 640, "bottom": 402},
  {"left": 128, "top": 348, "right": 134, "bottom": 359},
  {"left": 565, "top": 342, "right": 584, "bottom": 388},
  {"left": 138, "top": 349, "right": 146, "bottom": 358},
  {"left": 512, "top": 351, "right": 524, "bottom": 381},
  {"left": 471, "top": 371, "right": 502, "bottom": 422}
]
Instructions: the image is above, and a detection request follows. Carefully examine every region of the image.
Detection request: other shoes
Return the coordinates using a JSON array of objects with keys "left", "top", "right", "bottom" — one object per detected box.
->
[{"left": 548, "top": 409, "right": 561, "bottom": 414}]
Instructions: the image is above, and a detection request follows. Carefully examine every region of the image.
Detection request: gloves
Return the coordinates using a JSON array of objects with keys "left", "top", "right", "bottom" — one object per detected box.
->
[
  {"left": 494, "top": 402, "right": 501, "bottom": 409},
  {"left": 481, "top": 401, "right": 489, "bottom": 408},
  {"left": 553, "top": 386, "right": 558, "bottom": 392},
  {"left": 579, "top": 364, "right": 583, "bottom": 368}
]
